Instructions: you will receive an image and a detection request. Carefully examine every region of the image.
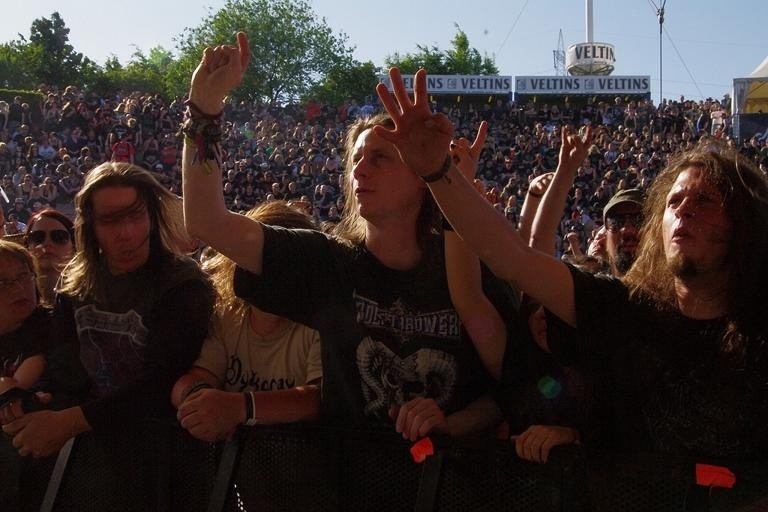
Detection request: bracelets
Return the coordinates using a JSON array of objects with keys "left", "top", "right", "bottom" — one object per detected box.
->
[
  {"left": 176, "top": 380, "right": 212, "bottom": 408},
  {"left": 529, "top": 190, "right": 543, "bottom": 200},
  {"left": 417, "top": 153, "right": 455, "bottom": 185},
  {"left": 182, "top": 96, "right": 222, "bottom": 174},
  {"left": 240, "top": 390, "right": 258, "bottom": 427}
]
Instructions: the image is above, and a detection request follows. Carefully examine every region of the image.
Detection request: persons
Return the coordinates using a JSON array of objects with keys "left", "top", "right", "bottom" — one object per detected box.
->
[
  {"left": 436, "top": 119, "right": 605, "bottom": 465},
  {"left": 367, "top": 66, "right": 765, "bottom": 511},
  {"left": 167, "top": 199, "right": 326, "bottom": 445},
  {"left": 179, "top": 30, "right": 521, "bottom": 508},
  {"left": 527, "top": 126, "right": 652, "bottom": 274},
  {"left": 25, "top": 209, "right": 75, "bottom": 306},
  {"left": 2, "top": 159, "right": 215, "bottom": 512},
  {"left": 0, "top": 73, "right": 766, "bottom": 276},
  {"left": 0, "top": 240, "right": 52, "bottom": 511}
]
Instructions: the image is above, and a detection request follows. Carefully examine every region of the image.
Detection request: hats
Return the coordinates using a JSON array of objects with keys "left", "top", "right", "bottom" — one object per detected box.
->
[{"left": 602, "top": 188, "right": 646, "bottom": 225}]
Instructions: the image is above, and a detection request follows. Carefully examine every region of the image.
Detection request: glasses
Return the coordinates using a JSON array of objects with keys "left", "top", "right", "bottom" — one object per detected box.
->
[
  {"left": 0, "top": 272, "right": 36, "bottom": 289},
  {"left": 605, "top": 213, "right": 645, "bottom": 233},
  {"left": 29, "top": 230, "right": 71, "bottom": 245}
]
